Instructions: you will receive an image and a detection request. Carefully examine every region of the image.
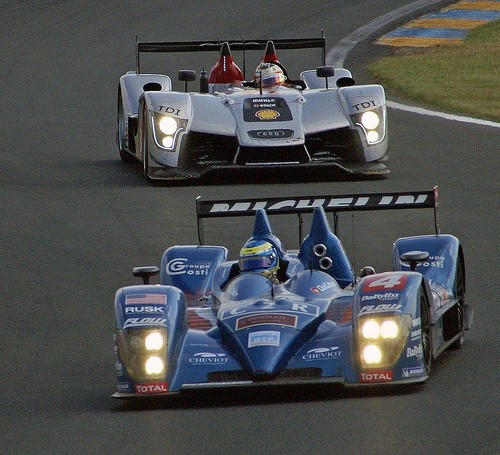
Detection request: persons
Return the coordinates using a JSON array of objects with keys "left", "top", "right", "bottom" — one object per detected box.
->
[
  {"left": 238, "top": 240, "right": 285, "bottom": 286},
  {"left": 255, "top": 63, "right": 285, "bottom": 88}
]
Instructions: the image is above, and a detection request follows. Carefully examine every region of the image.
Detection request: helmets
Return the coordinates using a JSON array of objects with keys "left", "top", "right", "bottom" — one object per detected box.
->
[
  {"left": 254, "top": 62, "right": 285, "bottom": 87},
  {"left": 238, "top": 239, "right": 280, "bottom": 272}
]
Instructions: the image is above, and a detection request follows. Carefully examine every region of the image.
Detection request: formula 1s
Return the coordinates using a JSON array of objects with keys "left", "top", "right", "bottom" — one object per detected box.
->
[
  {"left": 116, "top": 29, "right": 390, "bottom": 180},
  {"left": 110, "top": 186, "right": 473, "bottom": 399}
]
